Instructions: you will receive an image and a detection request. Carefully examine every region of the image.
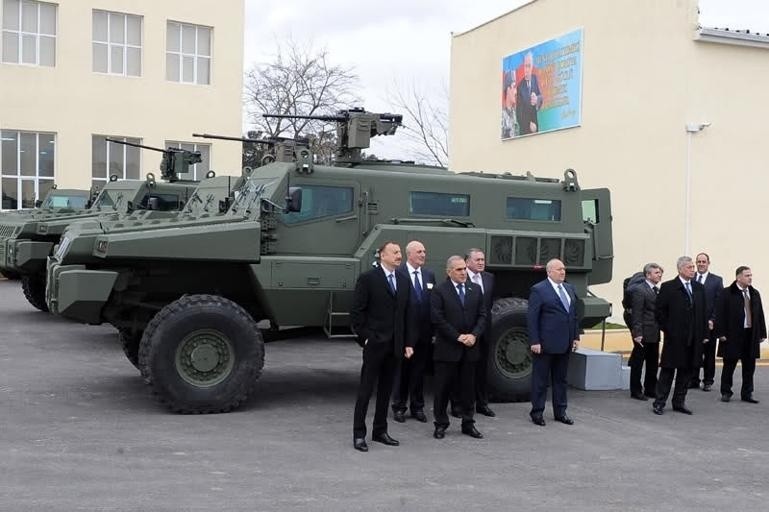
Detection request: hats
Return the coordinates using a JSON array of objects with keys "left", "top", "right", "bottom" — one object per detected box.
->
[{"left": 505, "top": 71, "right": 517, "bottom": 92}]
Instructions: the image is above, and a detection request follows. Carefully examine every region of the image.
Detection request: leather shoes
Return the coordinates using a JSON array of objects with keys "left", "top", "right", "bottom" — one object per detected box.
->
[
  {"left": 391, "top": 405, "right": 428, "bottom": 424},
  {"left": 450, "top": 408, "right": 463, "bottom": 418},
  {"left": 353, "top": 438, "right": 368, "bottom": 452},
  {"left": 630, "top": 391, "right": 658, "bottom": 401},
  {"left": 553, "top": 412, "right": 574, "bottom": 425},
  {"left": 721, "top": 393, "right": 731, "bottom": 402},
  {"left": 528, "top": 409, "right": 546, "bottom": 425},
  {"left": 740, "top": 396, "right": 760, "bottom": 404},
  {"left": 461, "top": 425, "right": 483, "bottom": 438},
  {"left": 433, "top": 426, "right": 445, "bottom": 439},
  {"left": 688, "top": 382, "right": 713, "bottom": 392},
  {"left": 371, "top": 432, "right": 400, "bottom": 446},
  {"left": 652, "top": 403, "right": 665, "bottom": 415},
  {"left": 671, "top": 404, "right": 692, "bottom": 416},
  {"left": 475, "top": 406, "right": 496, "bottom": 417}
]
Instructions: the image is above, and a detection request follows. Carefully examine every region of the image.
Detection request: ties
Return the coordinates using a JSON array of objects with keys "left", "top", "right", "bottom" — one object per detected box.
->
[
  {"left": 388, "top": 274, "right": 396, "bottom": 296},
  {"left": 698, "top": 276, "right": 703, "bottom": 283},
  {"left": 742, "top": 289, "right": 752, "bottom": 326},
  {"left": 557, "top": 285, "right": 570, "bottom": 313},
  {"left": 686, "top": 283, "right": 692, "bottom": 302},
  {"left": 472, "top": 275, "right": 480, "bottom": 286},
  {"left": 652, "top": 287, "right": 658, "bottom": 294},
  {"left": 457, "top": 284, "right": 465, "bottom": 307},
  {"left": 413, "top": 271, "right": 422, "bottom": 304}
]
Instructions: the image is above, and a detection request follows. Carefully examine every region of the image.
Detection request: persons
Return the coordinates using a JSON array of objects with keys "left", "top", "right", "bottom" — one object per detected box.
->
[
  {"left": 526, "top": 257, "right": 581, "bottom": 424},
  {"left": 351, "top": 241, "right": 414, "bottom": 451},
  {"left": 652, "top": 256, "right": 709, "bottom": 414},
  {"left": 450, "top": 248, "right": 498, "bottom": 418},
  {"left": 621, "top": 271, "right": 648, "bottom": 367},
  {"left": 392, "top": 240, "right": 436, "bottom": 422},
  {"left": 628, "top": 263, "right": 662, "bottom": 400},
  {"left": 430, "top": 254, "right": 487, "bottom": 441},
  {"left": 691, "top": 253, "right": 725, "bottom": 391},
  {"left": 503, "top": 71, "right": 521, "bottom": 138},
  {"left": 716, "top": 266, "right": 766, "bottom": 404},
  {"left": 516, "top": 51, "right": 543, "bottom": 135}
]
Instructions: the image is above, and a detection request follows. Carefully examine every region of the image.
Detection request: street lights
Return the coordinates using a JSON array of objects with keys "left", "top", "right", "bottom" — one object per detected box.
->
[{"left": 682, "top": 119, "right": 712, "bottom": 260}]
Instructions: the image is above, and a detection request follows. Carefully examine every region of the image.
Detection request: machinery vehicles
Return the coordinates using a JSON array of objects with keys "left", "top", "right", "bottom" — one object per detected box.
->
[{"left": 46, "top": 107, "right": 617, "bottom": 413}]
